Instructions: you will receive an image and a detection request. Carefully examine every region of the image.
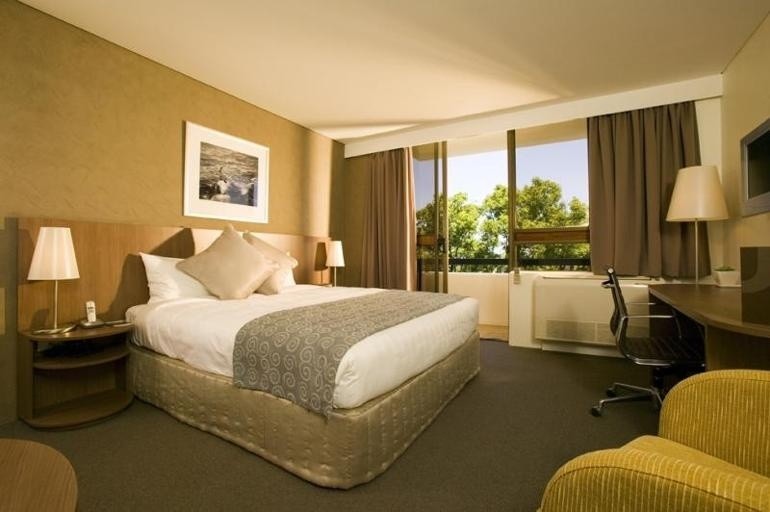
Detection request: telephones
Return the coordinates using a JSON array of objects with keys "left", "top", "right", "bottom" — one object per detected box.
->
[{"left": 80, "top": 299, "right": 104, "bottom": 329}]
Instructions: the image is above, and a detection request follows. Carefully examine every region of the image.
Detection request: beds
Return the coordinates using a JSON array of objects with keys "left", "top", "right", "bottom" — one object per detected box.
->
[{"left": 127, "top": 283, "right": 479, "bottom": 488}]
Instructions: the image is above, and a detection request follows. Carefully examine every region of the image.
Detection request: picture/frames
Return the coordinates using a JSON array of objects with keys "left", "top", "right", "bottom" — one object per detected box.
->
[{"left": 183, "top": 120, "right": 269, "bottom": 225}]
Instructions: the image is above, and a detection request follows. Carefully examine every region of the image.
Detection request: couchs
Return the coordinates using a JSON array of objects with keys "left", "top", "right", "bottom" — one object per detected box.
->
[{"left": 533, "top": 369, "right": 770, "bottom": 508}]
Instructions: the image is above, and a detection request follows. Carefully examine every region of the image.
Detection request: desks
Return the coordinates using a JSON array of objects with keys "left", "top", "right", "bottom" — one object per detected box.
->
[{"left": 648, "top": 282, "right": 768, "bottom": 397}]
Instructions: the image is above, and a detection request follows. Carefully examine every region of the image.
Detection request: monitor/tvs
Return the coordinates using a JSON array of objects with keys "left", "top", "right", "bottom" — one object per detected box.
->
[{"left": 738, "top": 116, "right": 770, "bottom": 217}]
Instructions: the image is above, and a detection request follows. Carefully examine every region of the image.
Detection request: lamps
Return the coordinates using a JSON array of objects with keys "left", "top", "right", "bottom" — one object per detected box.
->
[
  {"left": 326, "top": 241, "right": 343, "bottom": 286},
  {"left": 26, "top": 228, "right": 79, "bottom": 333},
  {"left": 665, "top": 165, "right": 726, "bottom": 285}
]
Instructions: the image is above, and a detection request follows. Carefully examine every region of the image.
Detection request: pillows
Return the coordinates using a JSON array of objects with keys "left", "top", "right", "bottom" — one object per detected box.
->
[
  {"left": 177, "top": 224, "right": 278, "bottom": 299},
  {"left": 139, "top": 250, "right": 215, "bottom": 303},
  {"left": 240, "top": 231, "right": 296, "bottom": 295}
]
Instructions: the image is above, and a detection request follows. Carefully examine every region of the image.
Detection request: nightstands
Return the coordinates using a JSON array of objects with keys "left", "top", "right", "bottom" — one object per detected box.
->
[{"left": 17, "top": 321, "right": 136, "bottom": 432}]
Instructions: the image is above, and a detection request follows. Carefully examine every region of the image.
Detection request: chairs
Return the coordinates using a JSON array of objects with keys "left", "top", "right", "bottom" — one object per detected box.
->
[{"left": 589, "top": 269, "right": 684, "bottom": 420}]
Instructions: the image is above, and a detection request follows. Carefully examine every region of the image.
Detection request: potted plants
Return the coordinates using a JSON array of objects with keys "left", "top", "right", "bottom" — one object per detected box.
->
[{"left": 713, "top": 264, "right": 741, "bottom": 287}]
